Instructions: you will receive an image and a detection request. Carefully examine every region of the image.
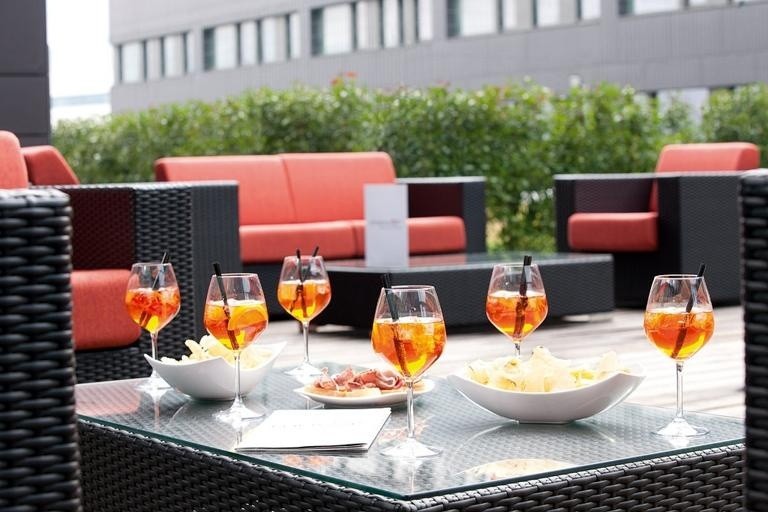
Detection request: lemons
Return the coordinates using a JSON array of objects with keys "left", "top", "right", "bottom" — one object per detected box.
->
[{"left": 228, "top": 304, "right": 267, "bottom": 331}]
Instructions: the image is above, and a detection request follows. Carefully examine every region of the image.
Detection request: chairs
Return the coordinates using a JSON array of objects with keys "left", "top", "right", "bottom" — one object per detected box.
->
[
  {"left": 552, "top": 142, "right": 760, "bottom": 310},
  {"left": 0, "top": 128, "right": 241, "bottom": 385}
]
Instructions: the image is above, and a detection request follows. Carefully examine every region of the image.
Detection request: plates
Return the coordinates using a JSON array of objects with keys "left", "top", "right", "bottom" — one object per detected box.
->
[
  {"left": 301, "top": 369, "right": 436, "bottom": 406},
  {"left": 144, "top": 336, "right": 289, "bottom": 402},
  {"left": 446, "top": 356, "right": 645, "bottom": 423}
]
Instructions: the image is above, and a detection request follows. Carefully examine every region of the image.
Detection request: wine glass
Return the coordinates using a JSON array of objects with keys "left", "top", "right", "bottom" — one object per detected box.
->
[
  {"left": 643, "top": 274, "right": 715, "bottom": 439},
  {"left": 371, "top": 287, "right": 451, "bottom": 454},
  {"left": 203, "top": 272, "right": 269, "bottom": 420},
  {"left": 277, "top": 255, "right": 331, "bottom": 376},
  {"left": 123, "top": 261, "right": 181, "bottom": 392},
  {"left": 485, "top": 264, "right": 549, "bottom": 359}
]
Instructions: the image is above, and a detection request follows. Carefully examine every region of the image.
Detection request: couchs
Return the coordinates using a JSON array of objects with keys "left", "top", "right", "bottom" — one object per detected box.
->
[{"left": 152, "top": 151, "right": 488, "bottom": 320}]
